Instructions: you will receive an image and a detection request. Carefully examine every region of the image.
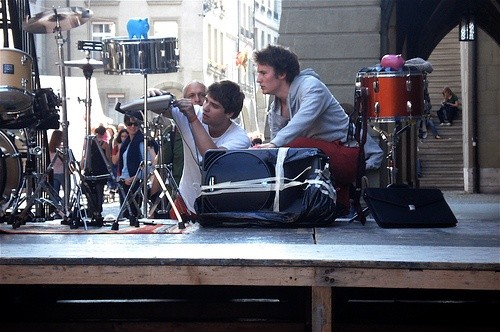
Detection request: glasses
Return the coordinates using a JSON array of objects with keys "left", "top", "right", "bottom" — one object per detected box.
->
[{"left": 125, "top": 121, "right": 138, "bottom": 126}]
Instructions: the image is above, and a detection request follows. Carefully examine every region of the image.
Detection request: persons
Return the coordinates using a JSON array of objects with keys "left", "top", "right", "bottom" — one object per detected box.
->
[
  {"left": 81, "top": 122, "right": 130, "bottom": 212},
  {"left": 157, "top": 79, "right": 210, "bottom": 208},
  {"left": 436, "top": 87, "right": 458, "bottom": 126},
  {"left": 417, "top": 112, "right": 440, "bottom": 139},
  {"left": 116, "top": 111, "right": 153, "bottom": 218},
  {"left": 49, "top": 130, "right": 76, "bottom": 218},
  {"left": 141, "top": 80, "right": 250, "bottom": 220},
  {"left": 339, "top": 103, "right": 384, "bottom": 171},
  {"left": 253, "top": 44, "right": 363, "bottom": 148}
]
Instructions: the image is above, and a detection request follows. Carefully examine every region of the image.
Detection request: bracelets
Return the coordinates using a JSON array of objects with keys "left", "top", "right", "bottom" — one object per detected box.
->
[{"left": 186, "top": 115, "right": 198, "bottom": 122}]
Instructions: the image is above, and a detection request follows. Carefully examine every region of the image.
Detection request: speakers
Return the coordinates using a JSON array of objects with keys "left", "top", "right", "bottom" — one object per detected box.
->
[{"left": 193, "top": 148, "right": 336, "bottom": 228}]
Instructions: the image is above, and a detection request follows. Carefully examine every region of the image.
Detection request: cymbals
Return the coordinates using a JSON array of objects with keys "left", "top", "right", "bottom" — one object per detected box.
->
[
  {"left": 55, "top": 58, "right": 103, "bottom": 70},
  {"left": 22, "top": 6, "right": 94, "bottom": 34}
]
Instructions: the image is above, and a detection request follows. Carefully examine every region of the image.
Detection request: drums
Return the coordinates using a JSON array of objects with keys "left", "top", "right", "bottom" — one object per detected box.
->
[
  {"left": 0, "top": 47, "right": 37, "bottom": 114},
  {"left": 0, "top": 88, "right": 40, "bottom": 129},
  {"left": 355, "top": 73, "right": 426, "bottom": 123},
  {"left": 103, "top": 36, "right": 180, "bottom": 76},
  {"left": 0, "top": 130, "right": 24, "bottom": 217},
  {"left": 27, "top": 87, "right": 62, "bottom": 129}
]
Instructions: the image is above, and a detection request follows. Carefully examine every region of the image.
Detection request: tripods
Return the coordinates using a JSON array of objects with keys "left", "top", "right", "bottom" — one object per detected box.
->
[
  {"left": 349, "top": 121, "right": 412, "bottom": 224},
  {"left": 7, "top": 8, "right": 190, "bottom": 231}
]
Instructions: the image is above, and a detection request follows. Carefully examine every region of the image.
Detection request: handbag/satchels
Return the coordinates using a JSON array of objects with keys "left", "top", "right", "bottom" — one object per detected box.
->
[{"left": 363, "top": 183, "right": 457, "bottom": 228}]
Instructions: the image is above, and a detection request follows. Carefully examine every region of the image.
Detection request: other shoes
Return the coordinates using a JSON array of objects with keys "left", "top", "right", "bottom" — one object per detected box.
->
[
  {"left": 440, "top": 123, "right": 451, "bottom": 126},
  {"left": 435, "top": 135, "right": 441, "bottom": 139},
  {"left": 423, "top": 132, "right": 428, "bottom": 139}
]
